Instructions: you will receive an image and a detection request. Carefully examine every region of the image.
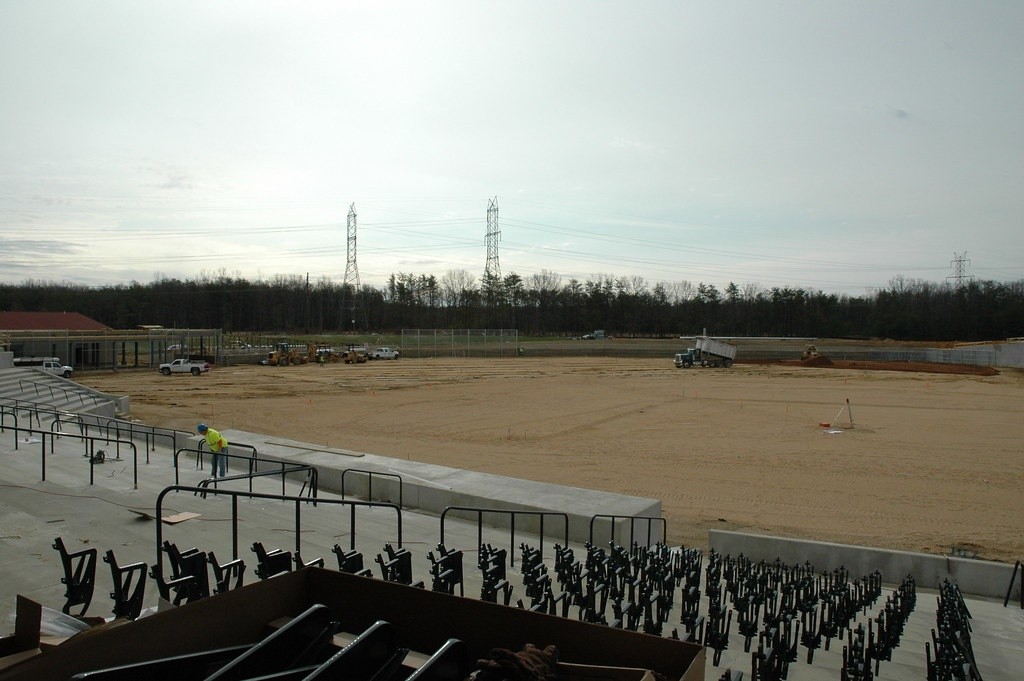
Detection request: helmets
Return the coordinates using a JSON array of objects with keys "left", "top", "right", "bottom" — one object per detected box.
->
[{"left": 198, "top": 424, "right": 206, "bottom": 432}]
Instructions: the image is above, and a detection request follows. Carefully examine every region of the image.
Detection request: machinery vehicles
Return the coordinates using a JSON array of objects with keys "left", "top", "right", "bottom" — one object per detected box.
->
[
  {"left": 800, "top": 344, "right": 819, "bottom": 361},
  {"left": 266, "top": 342, "right": 302, "bottom": 367}
]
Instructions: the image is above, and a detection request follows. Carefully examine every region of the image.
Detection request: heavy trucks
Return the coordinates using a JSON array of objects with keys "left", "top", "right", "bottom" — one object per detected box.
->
[{"left": 673, "top": 336, "right": 737, "bottom": 369}]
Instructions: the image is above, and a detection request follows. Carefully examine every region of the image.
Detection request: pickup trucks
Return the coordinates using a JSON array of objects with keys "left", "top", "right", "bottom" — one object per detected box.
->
[
  {"left": 13, "top": 356, "right": 74, "bottom": 379},
  {"left": 158, "top": 358, "right": 213, "bottom": 376}
]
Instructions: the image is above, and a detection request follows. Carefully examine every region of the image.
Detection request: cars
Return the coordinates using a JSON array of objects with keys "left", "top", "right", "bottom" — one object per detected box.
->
[
  {"left": 165, "top": 344, "right": 188, "bottom": 350},
  {"left": 311, "top": 343, "right": 373, "bottom": 364},
  {"left": 372, "top": 348, "right": 400, "bottom": 361},
  {"left": 566, "top": 329, "right": 614, "bottom": 340}
]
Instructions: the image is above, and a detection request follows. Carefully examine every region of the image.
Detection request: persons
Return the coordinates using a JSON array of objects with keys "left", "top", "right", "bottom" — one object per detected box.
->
[
  {"left": 198, "top": 424, "right": 227, "bottom": 480},
  {"left": 320, "top": 354, "right": 325, "bottom": 367},
  {"left": 520, "top": 346, "right": 525, "bottom": 356}
]
